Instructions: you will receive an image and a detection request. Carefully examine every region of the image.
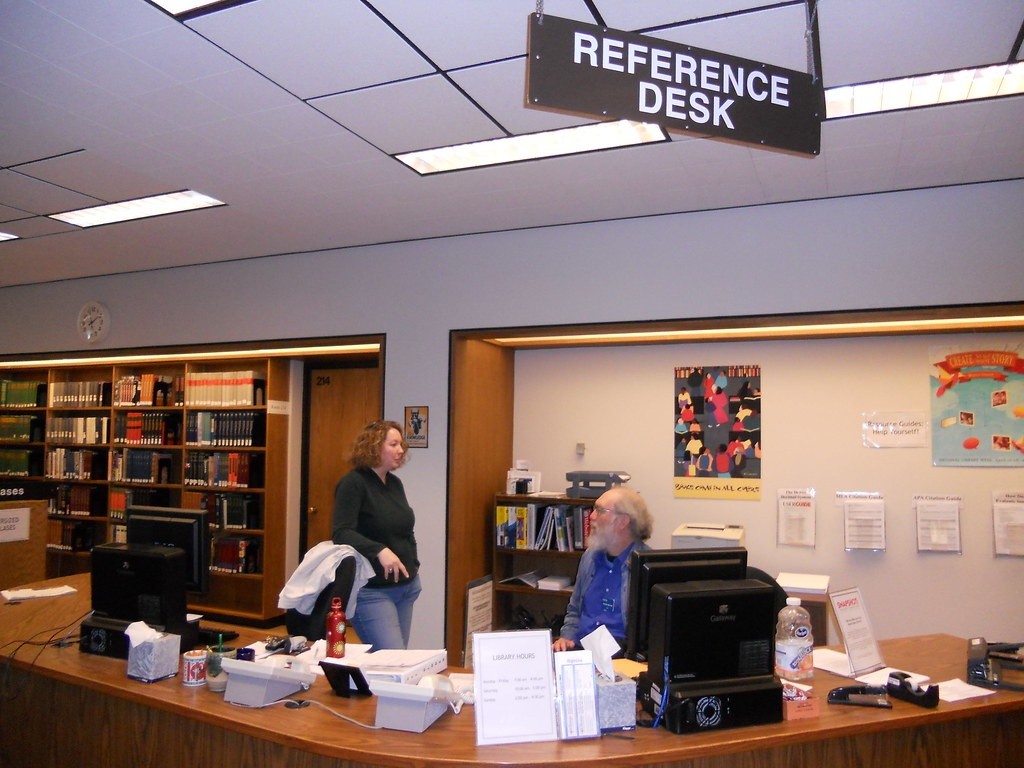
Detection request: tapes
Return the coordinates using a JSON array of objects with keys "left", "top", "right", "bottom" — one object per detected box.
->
[{"left": 905, "top": 678, "right": 919, "bottom": 691}]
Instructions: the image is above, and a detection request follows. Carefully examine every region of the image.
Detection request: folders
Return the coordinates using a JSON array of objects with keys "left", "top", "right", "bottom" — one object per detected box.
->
[{"left": 364, "top": 649, "right": 447, "bottom": 685}]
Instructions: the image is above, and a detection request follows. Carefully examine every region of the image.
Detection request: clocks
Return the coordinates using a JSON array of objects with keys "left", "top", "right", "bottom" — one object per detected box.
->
[{"left": 77, "top": 301, "right": 110, "bottom": 343}]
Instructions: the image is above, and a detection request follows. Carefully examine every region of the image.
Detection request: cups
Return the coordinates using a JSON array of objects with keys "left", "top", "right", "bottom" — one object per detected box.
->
[
  {"left": 236, "top": 649, "right": 256, "bottom": 662},
  {"left": 206, "top": 646, "right": 237, "bottom": 692},
  {"left": 183, "top": 650, "right": 207, "bottom": 686}
]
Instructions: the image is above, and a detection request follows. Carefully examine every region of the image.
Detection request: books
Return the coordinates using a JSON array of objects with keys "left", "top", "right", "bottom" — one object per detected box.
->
[
  {"left": 496, "top": 503, "right": 594, "bottom": 552},
  {"left": 0, "top": 370, "right": 266, "bottom": 573},
  {"left": 776, "top": 573, "right": 831, "bottom": 594}
]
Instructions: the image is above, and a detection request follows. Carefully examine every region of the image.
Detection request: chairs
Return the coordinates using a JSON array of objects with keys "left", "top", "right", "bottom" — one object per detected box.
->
[{"left": 274, "top": 544, "right": 376, "bottom": 641}]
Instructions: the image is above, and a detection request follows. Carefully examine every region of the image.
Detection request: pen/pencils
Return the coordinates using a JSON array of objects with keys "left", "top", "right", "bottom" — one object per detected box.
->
[
  {"left": 218, "top": 634, "right": 223, "bottom": 652},
  {"left": 205, "top": 646, "right": 213, "bottom": 653},
  {"left": 687, "top": 526, "right": 725, "bottom": 531}
]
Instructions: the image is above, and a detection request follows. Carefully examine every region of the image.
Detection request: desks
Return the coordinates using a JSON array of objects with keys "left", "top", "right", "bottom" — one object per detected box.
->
[
  {"left": 788, "top": 593, "right": 830, "bottom": 646},
  {"left": 1, "top": 571, "right": 1023, "bottom": 767}
]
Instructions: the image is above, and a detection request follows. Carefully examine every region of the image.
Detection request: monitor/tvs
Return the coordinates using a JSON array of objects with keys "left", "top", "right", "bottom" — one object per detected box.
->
[
  {"left": 127, "top": 505, "right": 211, "bottom": 597},
  {"left": 625, "top": 546, "right": 747, "bottom": 663}
]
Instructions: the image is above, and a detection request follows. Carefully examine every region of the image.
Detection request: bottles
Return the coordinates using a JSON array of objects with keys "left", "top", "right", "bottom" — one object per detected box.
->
[
  {"left": 326, "top": 597, "right": 345, "bottom": 658},
  {"left": 774, "top": 597, "right": 814, "bottom": 695}
]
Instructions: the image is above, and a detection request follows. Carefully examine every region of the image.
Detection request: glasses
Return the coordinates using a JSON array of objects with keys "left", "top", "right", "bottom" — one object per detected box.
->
[{"left": 592, "top": 505, "right": 632, "bottom": 525}]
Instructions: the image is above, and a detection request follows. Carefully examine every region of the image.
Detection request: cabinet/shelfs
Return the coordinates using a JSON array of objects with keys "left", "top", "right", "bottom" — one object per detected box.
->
[
  {"left": 0, "top": 357, "right": 286, "bottom": 631},
  {"left": 491, "top": 492, "right": 595, "bottom": 645}
]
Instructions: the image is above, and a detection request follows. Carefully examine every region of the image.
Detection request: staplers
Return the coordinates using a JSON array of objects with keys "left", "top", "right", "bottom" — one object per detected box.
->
[{"left": 827, "top": 685, "right": 892, "bottom": 708}]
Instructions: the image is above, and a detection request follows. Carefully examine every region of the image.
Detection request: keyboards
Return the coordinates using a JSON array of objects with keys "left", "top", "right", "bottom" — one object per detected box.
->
[{"left": 198, "top": 626, "right": 239, "bottom": 646}]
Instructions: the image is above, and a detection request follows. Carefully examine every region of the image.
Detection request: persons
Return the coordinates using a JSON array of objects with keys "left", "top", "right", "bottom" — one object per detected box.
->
[
  {"left": 333, "top": 420, "right": 422, "bottom": 652},
  {"left": 551, "top": 487, "right": 652, "bottom": 658}
]
